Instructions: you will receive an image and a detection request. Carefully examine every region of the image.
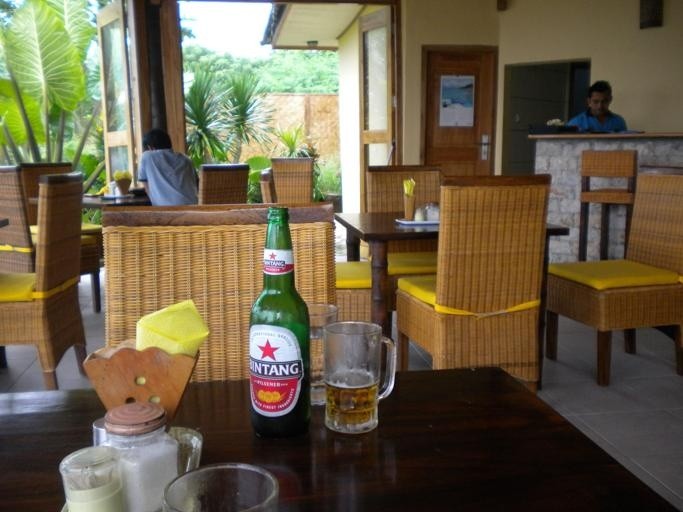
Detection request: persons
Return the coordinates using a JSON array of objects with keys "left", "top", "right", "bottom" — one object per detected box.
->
[
  {"left": 135, "top": 127, "right": 198, "bottom": 208},
  {"left": 543, "top": 77, "right": 629, "bottom": 132}
]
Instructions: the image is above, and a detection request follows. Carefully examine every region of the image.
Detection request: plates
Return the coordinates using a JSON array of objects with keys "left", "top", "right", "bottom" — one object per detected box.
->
[
  {"left": 588, "top": 129, "right": 644, "bottom": 135},
  {"left": 526, "top": 123, "right": 577, "bottom": 135}
]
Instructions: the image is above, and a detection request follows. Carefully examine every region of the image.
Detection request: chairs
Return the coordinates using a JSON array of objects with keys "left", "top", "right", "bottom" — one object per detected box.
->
[
  {"left": 196, "top": 164, "right": 249, "bottom": 203},
  {"left": 545, "top": 174, "right": 682, "bottom": 386},
  {"left": 257, "top": 169, "right": 372, "bottom": 322},
  {"left": 1, "top": 172, "right": 88, "bottom": 390},
  {"left": 576, "top": 147, "right": 638, "bottom": 261},
  {"left": 362, "top": 163, "right": 437, "bottom": 309},
  {"left": 395, "top": 172, "right": 551, "bottom": 386},
  {"left": 259, "top": 167, "right": 276, "bottom": 204},
  {"left": 100, "top": 200, "right": 337, "bottom": 383},
  {"left": 0, "top": 166, "right": 101, "bottom": 314},
  {"left": 270, "top": 155, "right": 314, "bottom": 203},
  {"left": 19, "top": 160, "right": 104, "bottom": 258}
]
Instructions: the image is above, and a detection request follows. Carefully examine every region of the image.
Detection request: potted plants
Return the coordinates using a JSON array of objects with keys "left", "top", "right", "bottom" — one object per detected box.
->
[{"left": 113, "top": 171, "right": 134, "bottom": 194}]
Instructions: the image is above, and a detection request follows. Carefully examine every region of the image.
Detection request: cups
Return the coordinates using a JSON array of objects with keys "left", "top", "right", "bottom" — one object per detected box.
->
[
  {"left": 300, "top": 299, "right": 339, "bottom": 409},
  {"left": 92, "top": 417, "right": 201, "bottom": 476},
  {"left": 319, "top": 318, "right": 397, "bottom": 436},
  {"left": 403, "top": 193, "right": 416, "bottom": 221},
  {"left": 160, "top": 464, "right": 277, "bottom": 512}
]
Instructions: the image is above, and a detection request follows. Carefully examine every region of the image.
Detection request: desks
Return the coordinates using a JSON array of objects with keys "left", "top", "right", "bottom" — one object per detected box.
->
[{"left": 0, "top": 363, "right": 682, "bottom": 509}]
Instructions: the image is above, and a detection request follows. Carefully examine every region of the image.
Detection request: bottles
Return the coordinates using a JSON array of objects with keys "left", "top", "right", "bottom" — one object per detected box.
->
[
  {"left": 90, "top": 403, "right": 176, "bottom": 512},
  {"left": 110, "top": 181, "right": 120, "bottom": 195},
  {"left": 247, "top": 206, "right": 312, "bottom": 439},
  {"left": 57, "top": 443, "right": 123, "bottom": 512},
  {"left": 423, "top": 201, "right": 438, "bottom": 220},
  {"left": 413, "top": 206, "right": 425, "bottom": 221}
]
